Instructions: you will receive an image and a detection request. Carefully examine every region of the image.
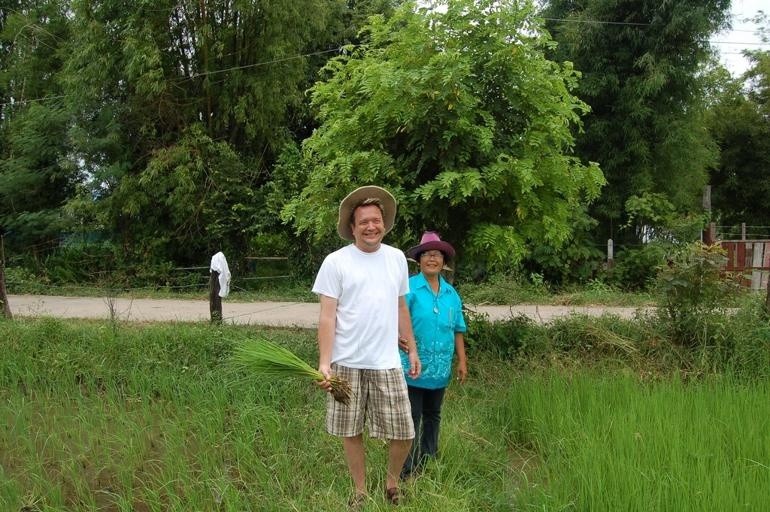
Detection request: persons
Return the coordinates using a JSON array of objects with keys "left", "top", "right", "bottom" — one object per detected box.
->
[
  {"left": 310, "top": 182, "right": 421, "bottom": 508},
  {"left": 398, "top": 227, "right": 469, "bottom": 486}
]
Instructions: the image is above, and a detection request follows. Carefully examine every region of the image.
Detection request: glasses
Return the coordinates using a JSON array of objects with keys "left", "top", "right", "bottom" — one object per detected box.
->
[{"left": 421, "top": 252, "right": 441, "bottom": 257}]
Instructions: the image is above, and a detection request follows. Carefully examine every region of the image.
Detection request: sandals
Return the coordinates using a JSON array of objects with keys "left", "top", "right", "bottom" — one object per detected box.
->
[
  {"left": 349, "top": 494, "right": 367, "bottom": 508},
  {"left": 385, "top": 486, "right": 406, "bottom": 505}
]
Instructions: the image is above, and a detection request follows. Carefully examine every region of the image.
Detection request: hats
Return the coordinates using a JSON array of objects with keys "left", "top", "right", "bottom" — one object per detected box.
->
[
  {"left": 337, "top": 186, "right": 397, "bottom": 241},
  {"left": 404, "top": 231, "right": 456, "bottom": 261}
]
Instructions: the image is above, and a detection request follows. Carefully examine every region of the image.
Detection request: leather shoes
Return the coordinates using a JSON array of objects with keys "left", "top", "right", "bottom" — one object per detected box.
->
[{"left": 399, "top": 468, "right": 417, "bottom": 481}]
